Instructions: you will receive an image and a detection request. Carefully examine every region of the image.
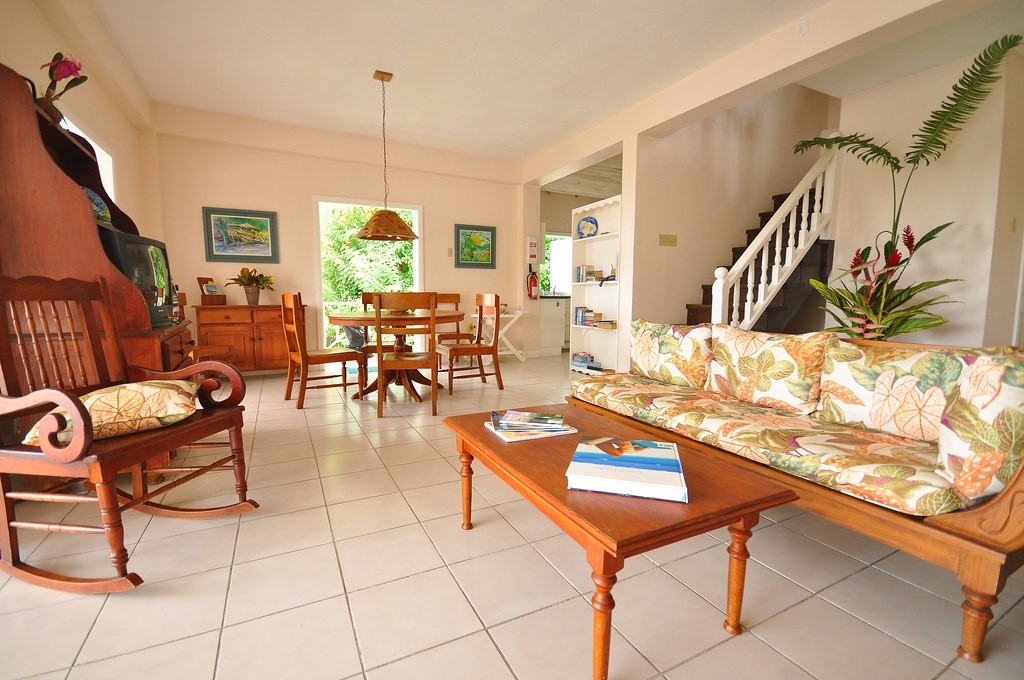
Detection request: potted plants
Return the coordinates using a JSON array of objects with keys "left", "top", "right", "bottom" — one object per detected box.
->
[{"left": 225, "top": 267, "right": 275, "bottom": 304}]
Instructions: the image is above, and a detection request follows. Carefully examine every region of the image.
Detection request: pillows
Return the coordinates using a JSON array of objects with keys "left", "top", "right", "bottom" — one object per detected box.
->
[{"left": 21, "top": 377, "right": 197, "bottom": 444}]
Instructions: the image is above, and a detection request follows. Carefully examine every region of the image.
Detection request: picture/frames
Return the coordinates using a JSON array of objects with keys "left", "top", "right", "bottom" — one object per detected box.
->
[
  {"left": 454, "top": 224, "right": 496, "bottom": 269},
  {"left": 202, "top": 206, "right": 280, "bottom": 264}
]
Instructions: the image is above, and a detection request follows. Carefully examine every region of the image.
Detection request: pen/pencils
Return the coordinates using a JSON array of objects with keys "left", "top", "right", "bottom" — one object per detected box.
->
[{"left": 611, "top": 264, "right": 613, "bottom": 268}]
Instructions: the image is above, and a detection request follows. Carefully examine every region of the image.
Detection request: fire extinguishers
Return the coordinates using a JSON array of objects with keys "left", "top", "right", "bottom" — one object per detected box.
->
[{"left": 527, "top": 272, "right": 539, "bottom": 300}]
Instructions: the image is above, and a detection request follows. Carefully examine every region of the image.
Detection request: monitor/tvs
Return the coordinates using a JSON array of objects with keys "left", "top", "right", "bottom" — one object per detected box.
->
[{"left": 98, "top": 229, "right": 173, "bottom": 327}]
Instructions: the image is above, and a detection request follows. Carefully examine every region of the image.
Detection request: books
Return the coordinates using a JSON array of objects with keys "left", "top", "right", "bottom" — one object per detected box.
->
[
  {"left": 565, "top": 436, "right": 688, "bottom": 505},
  {"left": 484, "top": 409, "right": 578, "bottom": 443},
  {"left": 575, "top": 265, "right": 603, "bottom": 326}
]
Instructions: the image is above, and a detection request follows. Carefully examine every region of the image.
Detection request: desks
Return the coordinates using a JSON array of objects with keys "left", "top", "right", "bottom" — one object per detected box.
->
[
  {"left": 470, "top": 310, "right": 529, "bottom": 362},
  {"left": 329, "top": 311, "right": 465, "bottom": 402}
]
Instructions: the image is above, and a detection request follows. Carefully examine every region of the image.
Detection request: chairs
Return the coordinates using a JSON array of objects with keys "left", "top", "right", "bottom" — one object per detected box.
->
[
  {"left": 281, "top": 293, "right": 364, "bottom": 409},
  {"left": 373, "top": 291, "right": 438, "bottom": 418},
  {"left": 435, "top": 294, "right": 503, "bottom": 395},
  {"left": 0, "top": 271, "right": 259, "bottom": 595},
  {"left": 428, "top": 293, "right": 460, "bottom": 369},
  {"left": 361, "top": 292, "right": 412, "bottom": 387}
]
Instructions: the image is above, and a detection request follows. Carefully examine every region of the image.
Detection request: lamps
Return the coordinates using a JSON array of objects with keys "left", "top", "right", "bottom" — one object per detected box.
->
[{"left": 354, "top": 70, "right": 418, "bottom": 241}]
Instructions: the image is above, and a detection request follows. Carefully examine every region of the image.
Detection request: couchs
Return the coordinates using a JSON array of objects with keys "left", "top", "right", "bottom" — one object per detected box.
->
[{"left": 564, "top": 320, "right": 1024, "bottom": 661}]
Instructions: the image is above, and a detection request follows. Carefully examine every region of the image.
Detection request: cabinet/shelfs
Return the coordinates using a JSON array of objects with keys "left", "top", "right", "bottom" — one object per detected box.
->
[
  {"left": 570, "top": 194, "right": 621, "bottom": 375},
  {"left": 117, "top": 327, "right": 192, "bottom": 372},
  {"left": 191, "top": 305, "right": 306, "bottom": 375}
]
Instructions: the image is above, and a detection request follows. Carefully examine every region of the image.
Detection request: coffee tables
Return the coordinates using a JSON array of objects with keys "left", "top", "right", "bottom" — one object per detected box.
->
[{"left": 442, "top": 404, "right": 800, "bottom": 680}]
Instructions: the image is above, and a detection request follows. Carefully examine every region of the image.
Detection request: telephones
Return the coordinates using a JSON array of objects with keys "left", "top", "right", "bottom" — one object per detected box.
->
[{"left": 604, "top": 275, "right": 615, "bottom": 281}]
